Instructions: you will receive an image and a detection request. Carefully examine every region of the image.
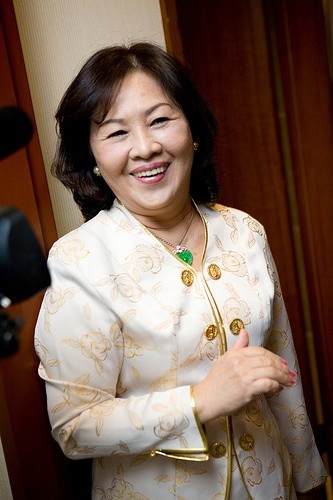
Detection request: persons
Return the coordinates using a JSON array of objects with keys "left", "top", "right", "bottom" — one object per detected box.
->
[{"left": 29, "top": 40, "right": 332, "bottom": 500}]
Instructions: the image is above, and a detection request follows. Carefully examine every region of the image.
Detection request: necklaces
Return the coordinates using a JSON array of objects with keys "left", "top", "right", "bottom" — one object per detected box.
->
[{"left": 150, "top": 208, "right": 197, "bottom": 267}]
